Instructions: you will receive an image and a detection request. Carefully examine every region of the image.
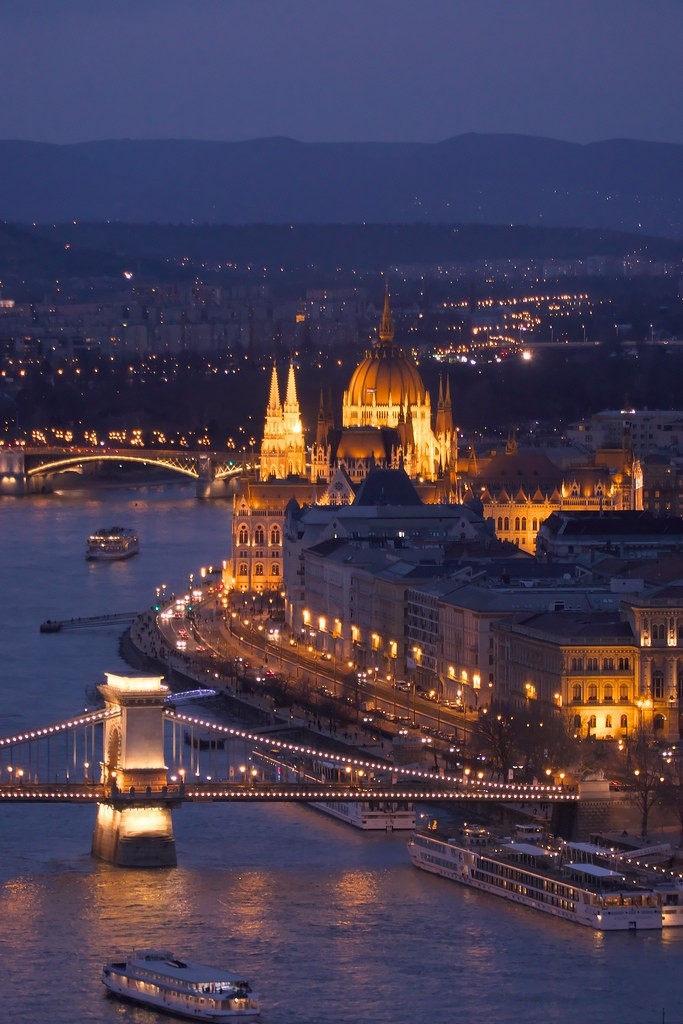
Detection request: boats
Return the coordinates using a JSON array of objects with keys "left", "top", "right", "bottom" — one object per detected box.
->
[
  {"left": 85, "top": 527, "right": 140, "bottom": 561},
  {"left": 101, "top": 948, "right": 261, "bottom": 1024}
]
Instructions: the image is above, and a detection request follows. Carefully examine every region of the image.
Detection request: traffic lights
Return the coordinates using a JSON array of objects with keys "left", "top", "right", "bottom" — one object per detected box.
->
[{"left": 156, "top": 607, "right": 159, "bottom": 611}]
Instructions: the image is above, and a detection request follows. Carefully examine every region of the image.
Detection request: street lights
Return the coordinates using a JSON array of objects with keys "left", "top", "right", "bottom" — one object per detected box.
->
[
  {"left": 245, "top": 621, "right": 253, "bottom": 655},
  {"left": 346, "top": 768, "right": 363, "bottom": 786},
  {"left": 328, "top": 654, "right": 336, "bottom": 693},
  {"left": 457, "top": 691, "right": 466, "bottom": 743},
  {"left": 387, "top": 673, "right": 396, "bottom": 715},
  {"left": 291, "top": 640, "right": 298, "bottom": 677},
  {"left": 309, "top": 647, "right": 317, "bottom": 686},
  {"left": 252, "top": 592, "right": 272, "bottom": 618},
  {"left": 179, "top": 770, "right": 184, "bottom": 783},
  {"left": 233, "top": 613, "right": 240, "bottom": 645},
  {"left": 430, "top": 688, "right": 440, "bottom": 731},
  {"left": 408, "top": 682, "right": 415, "bottom": 723},
  {"left": 368, "top": 668, "right": 377, "bottom": 710},
  {"left": 234, "top": 655, "right": 243, "bottom": 686},
  {"left": 348, "top": 660, "right": 357, "bottom": 700},
  {"left": 241, "top": 766, "right": 245, "bottom": 782},
  {"left": 259, "top": 626, "right": 267, "bottom": 663}
]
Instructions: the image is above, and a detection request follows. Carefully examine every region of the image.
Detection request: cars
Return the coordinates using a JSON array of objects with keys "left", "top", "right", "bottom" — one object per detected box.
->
[
  {"left": 173, "top": 613, "right": 181, "bottom": 619},
  {"left": 662, "top": 746, "right": 681, "bottom": 756},
  {"left": 321, "top": 654, "right": 332, "bottom": 661},
  {"left": 179, "top": 629, "right": 189, "bottom": 640},
  {"left": 314, "top": 681, "right": 464, "bottom": 745}
]
